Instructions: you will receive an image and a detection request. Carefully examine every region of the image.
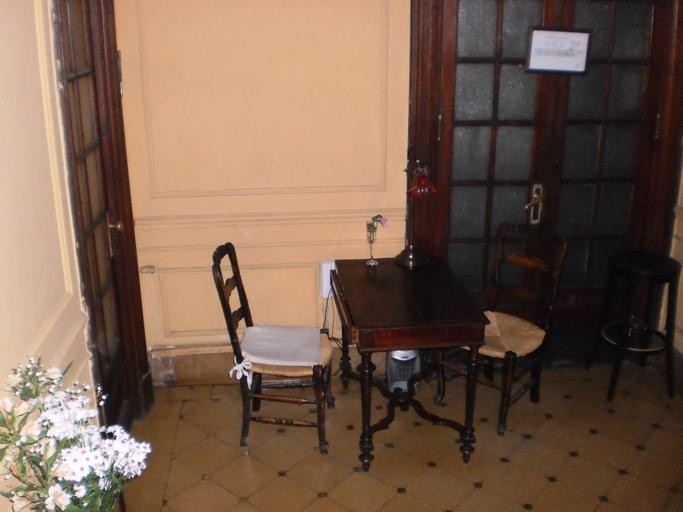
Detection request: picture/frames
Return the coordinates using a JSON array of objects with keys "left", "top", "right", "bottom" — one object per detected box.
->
[{"left": 525, "top": 26, "right": 592, "bottom": 75}]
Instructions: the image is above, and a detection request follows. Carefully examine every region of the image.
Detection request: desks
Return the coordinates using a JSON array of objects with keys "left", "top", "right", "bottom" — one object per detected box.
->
[{"left": 331, "top": 256, "right": 490, "bottom": 470}]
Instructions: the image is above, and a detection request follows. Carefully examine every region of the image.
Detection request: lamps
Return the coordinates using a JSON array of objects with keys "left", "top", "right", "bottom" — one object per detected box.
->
[{"left": 393, "top": 147, "right": 432, "bottom": 272}]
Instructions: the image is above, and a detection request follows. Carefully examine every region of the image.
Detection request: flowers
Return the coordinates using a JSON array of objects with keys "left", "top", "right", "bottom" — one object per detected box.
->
[
  {"left": 0, "top": 355, "right": 152, "bottom": 512},
  {"left": 367, "top": 215, "right": 389, "bottom": 260}
]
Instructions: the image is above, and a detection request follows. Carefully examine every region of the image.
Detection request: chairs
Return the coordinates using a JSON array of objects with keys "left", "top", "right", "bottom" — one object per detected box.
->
[
  {"left": 212, "top": 243, "right": 334, "bottom": 455},
  {"left": 434, "top": 224, "right": 569, "bottom": 436}
]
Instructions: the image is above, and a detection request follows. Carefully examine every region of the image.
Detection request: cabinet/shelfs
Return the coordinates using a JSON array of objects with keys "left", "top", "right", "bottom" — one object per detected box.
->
[{"left": 585, "top": 252, "right": 681, "bottom": 401}]
